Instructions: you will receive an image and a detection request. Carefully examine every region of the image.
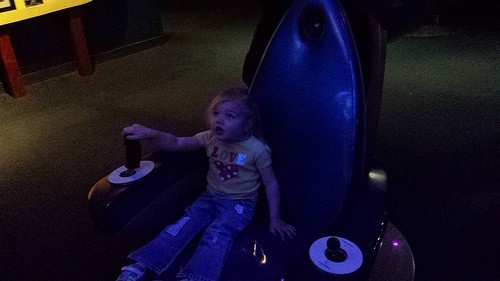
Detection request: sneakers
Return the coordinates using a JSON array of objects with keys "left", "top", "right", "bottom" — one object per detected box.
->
[{"left": 116, "top": 262, "right": 145, "bottom": 281}]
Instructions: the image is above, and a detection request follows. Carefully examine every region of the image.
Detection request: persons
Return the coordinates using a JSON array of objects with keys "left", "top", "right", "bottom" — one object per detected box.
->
[{"left": 115, "top": 86, "right": 298, "bottom": 281}]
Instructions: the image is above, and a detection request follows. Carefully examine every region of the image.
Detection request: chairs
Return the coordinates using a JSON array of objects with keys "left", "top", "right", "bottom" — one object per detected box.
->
[{"left": 85, "top": 0, "right": 396, "bottom": 281}]
